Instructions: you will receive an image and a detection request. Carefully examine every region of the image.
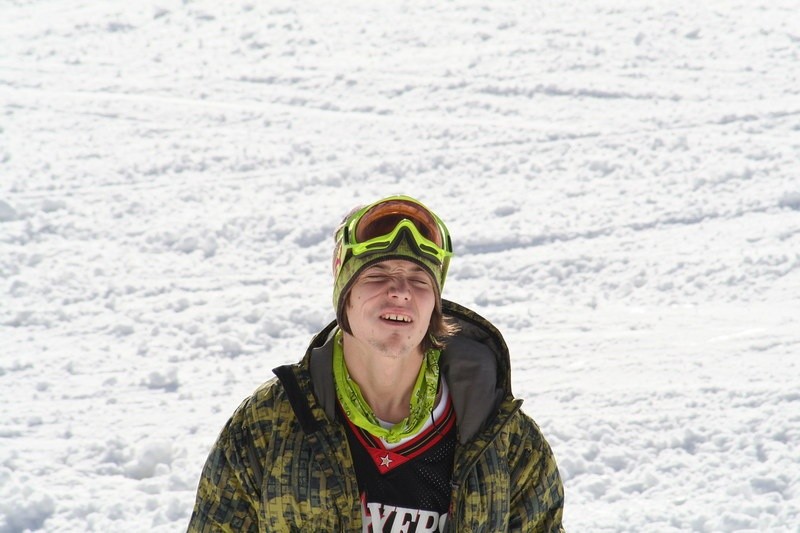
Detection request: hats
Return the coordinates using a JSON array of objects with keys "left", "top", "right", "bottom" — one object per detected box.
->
[{"left": 332, "top": 204, "right": 453, "bottom": 329}]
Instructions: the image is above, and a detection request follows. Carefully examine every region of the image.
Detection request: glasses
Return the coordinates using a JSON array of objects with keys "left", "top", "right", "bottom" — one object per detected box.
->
[{"left": 343, "top": 195, "right": 453, "bottom": 263}]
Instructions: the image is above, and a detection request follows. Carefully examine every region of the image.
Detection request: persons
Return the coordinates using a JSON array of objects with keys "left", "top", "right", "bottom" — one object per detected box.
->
[{"left": 185, "top": 194, "right": 565, "bottom": 533}]
